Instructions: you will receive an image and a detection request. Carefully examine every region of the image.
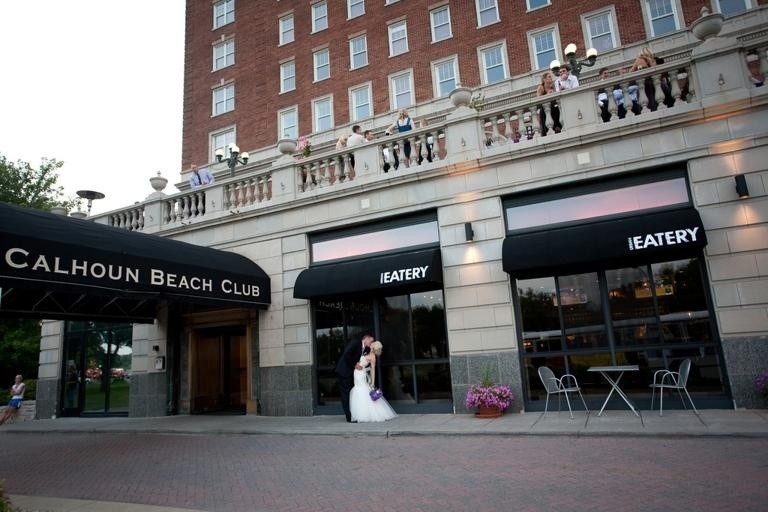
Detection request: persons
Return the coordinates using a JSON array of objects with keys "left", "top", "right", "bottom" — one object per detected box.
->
[
  {"left": 337, "top": 334, "right": 375, "bottom": 423},
  {"left": 536, "top": 73, "right": 563, "bottom": 137},
  {"left": 613, "top": 67, "right": 642, "bottom": 120},
  {"left": 628, "top": 45, "right": 674, "bottom": 113},
  {"left": 554, "top": 65, "right": 580, "bottom": 93},
  {"left": 335, "top": 106, "right": 434, "bottom": 182},
  {"left": 189, "top": 162, "right": 214, "bottom": 190},
  {"left": 597, "top": 68, "right": 617, "bottom": 123},
  {"left": 0, "top": 374, "right": 25, "bottom": 425},
  {"left": 349, "top": 340, "right": 398, "bottom": 423},
  {"left": 653, "top": 54, "right": 671, "bottom": 92}
]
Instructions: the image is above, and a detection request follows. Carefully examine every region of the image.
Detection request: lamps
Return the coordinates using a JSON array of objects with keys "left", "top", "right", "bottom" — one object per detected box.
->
[
  {"left": 465, "top": 222, "right": 474, "bottom": 243},
  {"left": 734, "top": 174, "right": 749, "bottom": 199}
]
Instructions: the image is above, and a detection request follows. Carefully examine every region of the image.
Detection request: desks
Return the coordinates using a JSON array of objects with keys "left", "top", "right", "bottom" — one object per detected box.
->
[{"left": 586, "top": 364, "right": 642, "bottom": 417}]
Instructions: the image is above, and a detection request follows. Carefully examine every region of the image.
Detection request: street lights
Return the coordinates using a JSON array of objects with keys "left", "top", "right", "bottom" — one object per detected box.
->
[
  {"left": 215, "top": 142, "right": 248, "bottom": 177},
  {"left": 548, "top": 43, "right": 598, "bottom": 81}
]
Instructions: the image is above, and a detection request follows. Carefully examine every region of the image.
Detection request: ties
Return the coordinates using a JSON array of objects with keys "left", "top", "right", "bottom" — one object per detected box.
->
[{"left": 197, "top": 174, "right": 201, "bottom": 184}]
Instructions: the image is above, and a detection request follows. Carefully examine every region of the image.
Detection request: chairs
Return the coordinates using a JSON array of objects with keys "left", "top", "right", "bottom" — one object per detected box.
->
[
  {"left": 537, "top": 365, "right": 590, "bottom": 418},
  {"left": 649, "top": 357, "right": 702, "bottom": 418}
]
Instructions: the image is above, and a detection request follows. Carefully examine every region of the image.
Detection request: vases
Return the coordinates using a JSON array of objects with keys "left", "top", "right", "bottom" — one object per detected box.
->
[{"left": 478, "top": 403, "right": 502, "bottom": 419}]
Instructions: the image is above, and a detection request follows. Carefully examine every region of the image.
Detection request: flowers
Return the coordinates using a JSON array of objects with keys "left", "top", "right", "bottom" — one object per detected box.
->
[{"left": 465, "top": 372, "right": 513, "bottom": 409}]
